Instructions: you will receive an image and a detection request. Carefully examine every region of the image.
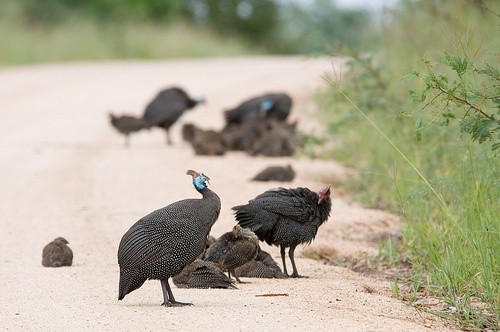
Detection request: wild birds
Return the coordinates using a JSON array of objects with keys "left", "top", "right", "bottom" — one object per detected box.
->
[
  {"left": 251, "top": 163, "right": 295, "bottom": 182},
  {"left": 41, "top": 236, "right": 73, "bottom": 268},
  {"left": 117, "top": 170, "right": 220, "bottom": 309},
  {"left": 181, "top": 93, "right": 303, "bottom": 157},
  {"left": 108, "top": 86, "right": 206, "bottom": 145},
  {"left": 231, "top": 184, "right": 333, "bottom": 279},
  {"left": 172, "top": 225, "right": 290, "bottom": 290}
]
